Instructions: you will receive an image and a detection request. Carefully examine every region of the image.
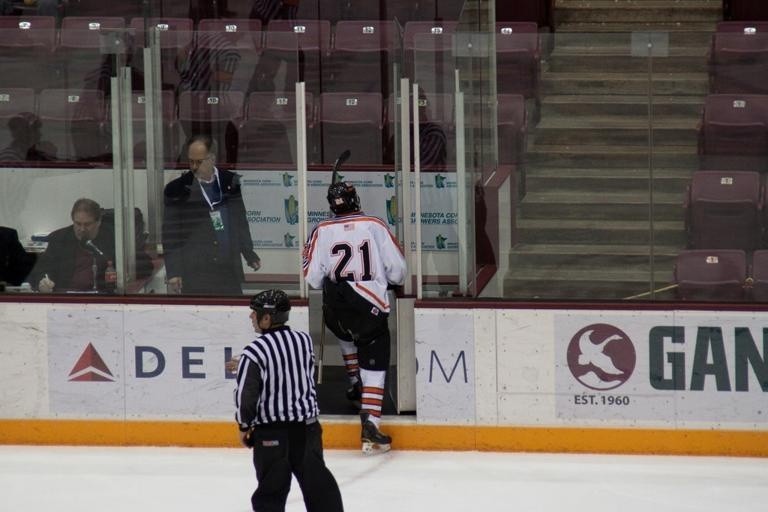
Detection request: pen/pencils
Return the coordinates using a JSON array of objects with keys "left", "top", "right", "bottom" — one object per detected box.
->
[{"left": 46, "top": 274, "right": 49, "bottom": 280}]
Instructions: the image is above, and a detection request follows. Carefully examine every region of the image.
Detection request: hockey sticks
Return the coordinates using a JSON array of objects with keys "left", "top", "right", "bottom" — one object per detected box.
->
[{"left": 317, "top": 149, "right": 351, "bottom": 386}]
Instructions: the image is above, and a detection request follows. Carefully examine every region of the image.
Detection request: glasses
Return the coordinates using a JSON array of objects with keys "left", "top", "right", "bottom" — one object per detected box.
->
[{"left": 188, "top": 157, "right": 211, "bottom": 166}]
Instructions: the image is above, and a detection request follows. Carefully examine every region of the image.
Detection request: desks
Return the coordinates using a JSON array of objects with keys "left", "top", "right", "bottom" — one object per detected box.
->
[{"left": 2, "top": 277, "right": 155, "bottom": 294}]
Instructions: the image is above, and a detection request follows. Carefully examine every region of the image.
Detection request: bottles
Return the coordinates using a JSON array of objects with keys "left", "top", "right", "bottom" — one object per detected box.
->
[{"left": 104, "top": 260, "right": 117, "bottom": 295}]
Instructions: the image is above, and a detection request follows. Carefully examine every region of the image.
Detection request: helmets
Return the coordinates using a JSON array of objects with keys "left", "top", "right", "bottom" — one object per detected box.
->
[
  {"left": 247, "top": 288, "right": 292, "bottom": 323},
  {"left": 326, "top": 179, "right": 362, "bottom": 215}
]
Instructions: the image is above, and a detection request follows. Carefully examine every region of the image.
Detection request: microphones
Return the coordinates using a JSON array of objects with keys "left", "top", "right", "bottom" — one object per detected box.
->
[{"left": 80, "top": 236, "right": 104, "bottom": 255}]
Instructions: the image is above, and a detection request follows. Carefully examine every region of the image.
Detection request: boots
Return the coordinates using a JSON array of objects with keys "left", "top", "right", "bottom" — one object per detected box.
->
[
  {"left": 359, "top": 411, "right": 392, "bottom": 444},
  {"left": 344, "top": 370, "right": 363, "bottom": 400}
]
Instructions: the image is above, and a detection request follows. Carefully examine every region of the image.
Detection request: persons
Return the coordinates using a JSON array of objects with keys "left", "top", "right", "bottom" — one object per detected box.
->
[
  {"left": 24, "top": 197, "right": 117, "bottom": 293},
  {"left": 233, "top": 289, "right": 344, "bottom": 512},
  {"left": 302, "top": 183, "right": 408, "bottom": 445},
  {"left": 382, "top": 91, "right": 447, "bottom": 174},
  {"left": 169, "top": 17, "right": 248, "bottom": 174},
  {"left": 161, "top": 135, "right": 261, "bottom": 296},
  {"left": 70, "top": 31, "right": 143, "bottom": 165},
  {"left": 0, "top": 112, "right": 49, "bottom": 162}
]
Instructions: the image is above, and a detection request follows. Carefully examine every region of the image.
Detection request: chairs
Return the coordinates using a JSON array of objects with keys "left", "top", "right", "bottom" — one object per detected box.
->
[{"left": 670, "top": 0, "right": 768, "bottom": 304}]
